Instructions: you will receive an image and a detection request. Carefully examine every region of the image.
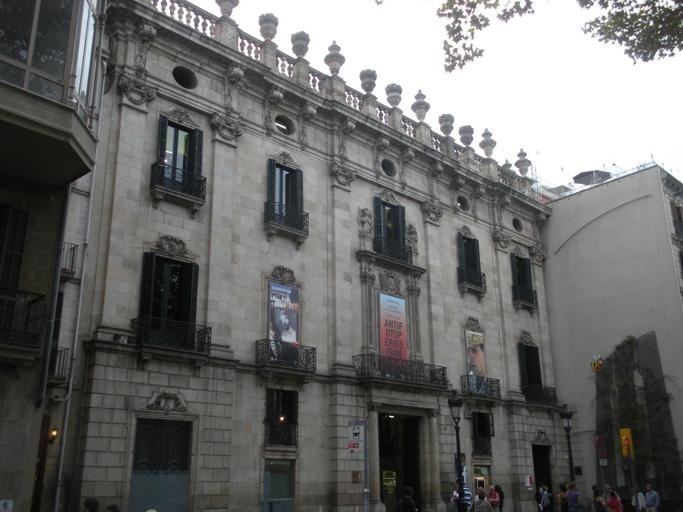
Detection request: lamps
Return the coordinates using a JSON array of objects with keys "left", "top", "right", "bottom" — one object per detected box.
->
[{"left": 48, "top": 425, "right": 58, "bottom": 443}]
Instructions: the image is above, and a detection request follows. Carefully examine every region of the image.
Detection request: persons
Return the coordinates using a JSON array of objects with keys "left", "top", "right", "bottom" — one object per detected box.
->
[
  {"left": 530, "top": 481, "right": 626, "bottom": 512},
  {"left": 447, "top": 483, "right": 504, "bottom": 512},
  {"left": 466, "top": 341, "right": 487, "bottom": 393},
  {"left": 629, "top": 486, "right": 646, "bottom": 512},
  {"left": 644, "top": 483, "right": 660, "bottom": 512},
  {"left": 279, "top": 310, "right": 296, "bottom": 343},
  {"left": 396, "top": 487, "right": 418, "bottom": 512}
]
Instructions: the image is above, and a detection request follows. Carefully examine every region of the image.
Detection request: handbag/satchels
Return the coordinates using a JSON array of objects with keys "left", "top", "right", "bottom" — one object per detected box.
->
[{"left": 539, "top": 504, "right": 543, "bottom": 511}]
